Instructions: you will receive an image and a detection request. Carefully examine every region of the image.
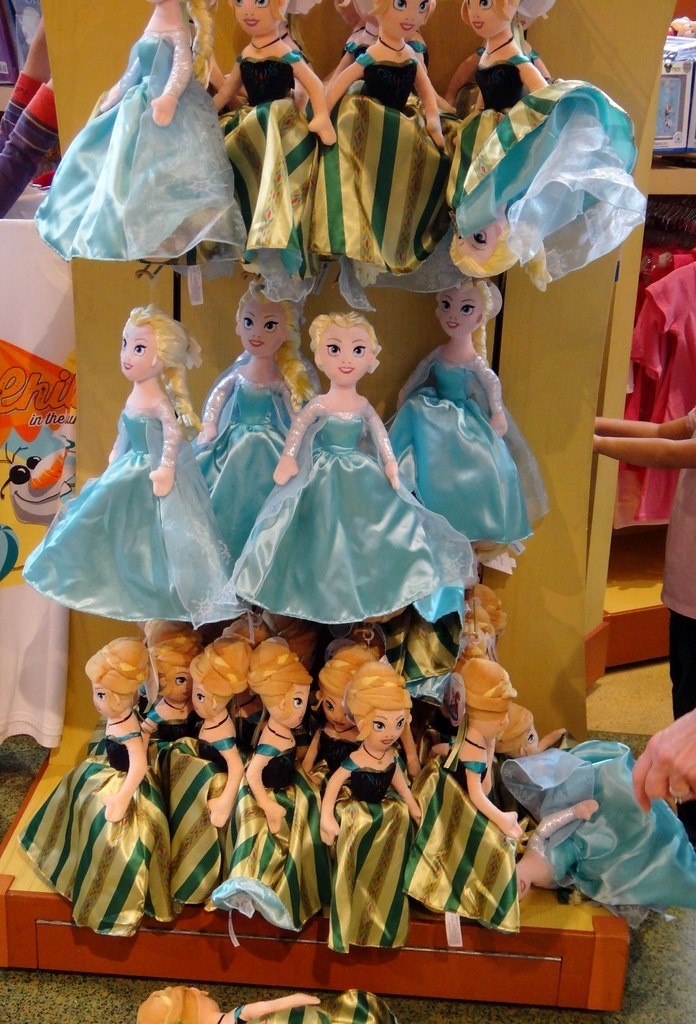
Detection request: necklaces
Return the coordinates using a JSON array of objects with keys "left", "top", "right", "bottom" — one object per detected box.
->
[
  {"left": 201, "top": 715, "right": 228, "bottom": 732},
  {"left": 332, "top": 724, "right": 356, "bottom": 738},
  {"left": 281, "top": 32, "right": 288, "bottom": 39},
  {"left": 465, "top": 738, "right": 497, "bottom": 764},
  {"left": 105, "top": 711, "right": 132, "bottom": 728},
  {"left": 235, "top": 695, "right": 257, "bottom": 710},
  {"left": 218, "top": 1013, "right": 225, "bottom": 1024},
  {"left": 354, "top": 26, "right": 365, "bottom": 33},
  {"left": 379, "top": 37, "right": 406, "bottom": 57},
  {"left": 365, "top": 29, "right": 378, "bottom": 41},
  {"left": 250, "top": 38, "right": 280, "bottom": 53},
  {"left": 486, "top": 37, "right": 513, "bottom": 59},
  {"left": 363, "top": 743, "right": 387, "bottom": 765},
  {"left": 163, "top": 698, "right": 187, "bottom": 715},
  {"left": 267, "top": 724, "right": 294, "bottom": 745}
]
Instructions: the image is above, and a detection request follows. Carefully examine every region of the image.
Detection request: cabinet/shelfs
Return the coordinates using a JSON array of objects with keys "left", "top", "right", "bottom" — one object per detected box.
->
[
  {"left": 578, "top": 36, "right": 695, "bottom": 695},
  {"left": 0, "top": 0, "right": 676, "bottom": 1008}
]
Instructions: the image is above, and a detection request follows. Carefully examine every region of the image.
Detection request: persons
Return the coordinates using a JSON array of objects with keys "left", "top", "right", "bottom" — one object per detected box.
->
[
  {"left": 596, "top": 404, "right": 696, "bottom": 850},
  {"left": 17, "top": 585, "right": 696, "bottom": 954},
  {"left": 0, "top": 15, "right": 59, "bottom": 219},
  {"left": 136, "top": 985, "right": 399, "bottom": 1024},
  {"left": 20, "top": 279, "right": 552, "bottom": 631},
  {"left": 33, "top": 0, "right": 646, "bottom": 315}
]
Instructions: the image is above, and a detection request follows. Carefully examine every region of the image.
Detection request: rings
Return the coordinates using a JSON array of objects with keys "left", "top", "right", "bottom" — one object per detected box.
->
[{"left": 669, "top": 787, "right": 690, "bottom": 797}]
